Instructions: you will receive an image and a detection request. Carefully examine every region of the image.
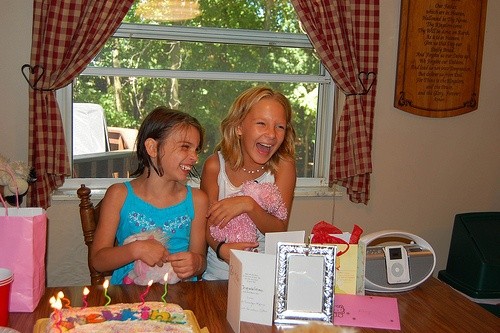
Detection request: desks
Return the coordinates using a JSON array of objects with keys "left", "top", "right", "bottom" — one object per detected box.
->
[{"left": 6, "top": 274, "right": 500, "bottom": 333}]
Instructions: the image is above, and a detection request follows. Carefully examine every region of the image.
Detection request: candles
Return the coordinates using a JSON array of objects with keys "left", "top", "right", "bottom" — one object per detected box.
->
[
  {"left": 103, "top": 280, "right": 111, "bottom": 308},
  {"left": 58, "top": 290, "right": 73, "bottom": 316},
  {"left": 81, "top": 286, "right": 90, "bottom": 310},
  {"left": 161, "top": 272, "right": 168, "bottom": 306},
  {"left": 49, "top": 297, "right": 63, "bottom": 333},
  {"left": 138, "top": 279, "right": 153, "bottom": 307}
]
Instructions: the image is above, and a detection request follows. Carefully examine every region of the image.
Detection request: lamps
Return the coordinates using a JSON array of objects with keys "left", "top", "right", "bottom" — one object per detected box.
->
[{"left": 135, "top": 0, "right": 203, "bottom": 22}]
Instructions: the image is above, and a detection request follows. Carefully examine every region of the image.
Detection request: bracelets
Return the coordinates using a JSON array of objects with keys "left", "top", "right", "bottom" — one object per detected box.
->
[{"left": 216, "top": 241, "right": 225, "bottom": 259}]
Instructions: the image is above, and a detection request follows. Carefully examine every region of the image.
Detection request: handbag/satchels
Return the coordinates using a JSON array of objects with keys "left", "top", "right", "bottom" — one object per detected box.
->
[{"left": 0, "top": 168, "right": 47, "bottom": 312}]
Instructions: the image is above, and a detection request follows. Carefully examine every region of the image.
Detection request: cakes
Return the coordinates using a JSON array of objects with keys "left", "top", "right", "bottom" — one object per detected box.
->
[{"left": 46, "top": 302, "right": 195, "bottom": 333}]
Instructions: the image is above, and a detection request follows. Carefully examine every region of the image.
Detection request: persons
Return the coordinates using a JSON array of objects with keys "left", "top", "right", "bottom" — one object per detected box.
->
[
  {"left": 200, "top": 85, "right": 297, "bottom": 281},
  {"left": 91, "top": 108, "right": 208, "bottom": 286}
]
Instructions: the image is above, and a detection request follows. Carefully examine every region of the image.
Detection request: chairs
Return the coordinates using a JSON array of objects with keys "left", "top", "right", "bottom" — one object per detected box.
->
[
  {"left": 436, "top": 212, "right": 500, "bottom": 317},
  {"left": 77, "top": 185, "right": 118, "bottom": 285}
]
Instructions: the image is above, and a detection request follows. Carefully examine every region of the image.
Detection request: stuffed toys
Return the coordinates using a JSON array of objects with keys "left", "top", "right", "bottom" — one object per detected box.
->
[{"left": 0, "top": 154, "right": 28, "bottom": 197}]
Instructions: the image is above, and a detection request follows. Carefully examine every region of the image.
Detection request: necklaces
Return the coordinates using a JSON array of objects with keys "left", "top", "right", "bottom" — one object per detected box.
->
[{"left": 241, "top": 166, "right": 264, "bottom": 174}]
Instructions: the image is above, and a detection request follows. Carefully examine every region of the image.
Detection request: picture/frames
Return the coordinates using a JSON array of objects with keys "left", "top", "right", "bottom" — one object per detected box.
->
[{"left": 274, "top": 242, "right": 337, "bottom": 325}]
